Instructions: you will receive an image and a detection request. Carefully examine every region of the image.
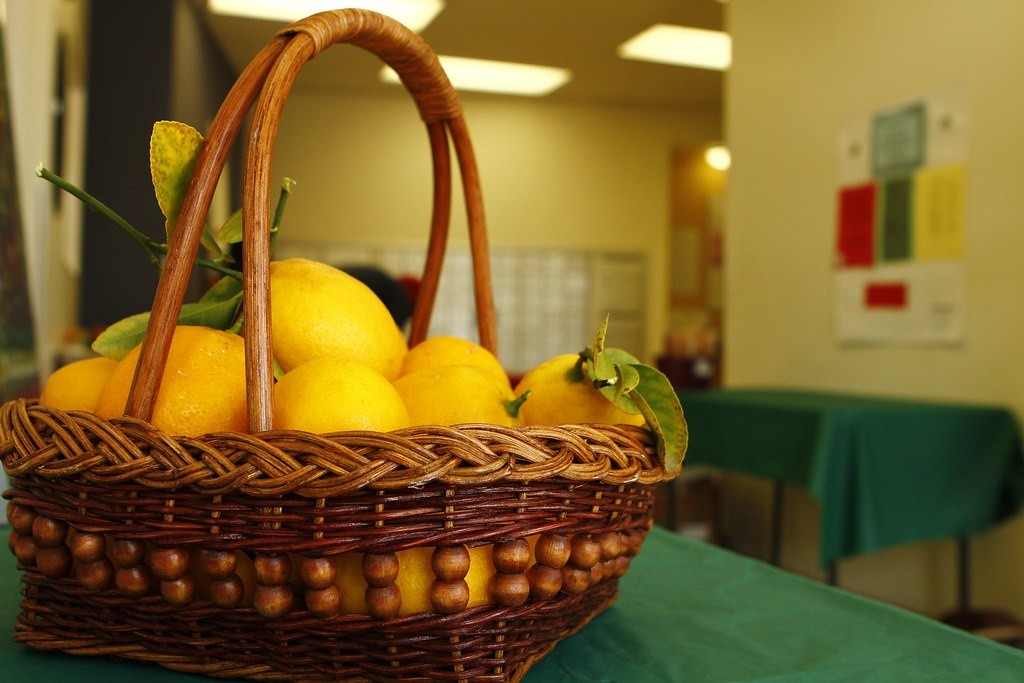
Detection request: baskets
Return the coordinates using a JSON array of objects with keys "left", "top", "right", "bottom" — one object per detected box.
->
[{"left": 0, "top": 4, "right": 692, "bottom": 683}]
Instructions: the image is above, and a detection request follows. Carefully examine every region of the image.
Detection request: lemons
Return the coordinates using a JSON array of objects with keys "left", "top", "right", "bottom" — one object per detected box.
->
[
  {"left": 406, "top": 337, "right": 510, "bottom": 390},
  {"left": 40, "top": 357, "right": 120, "bottom": 413},
  {"left": 98, "top": 326, "right": 248, "bottom": 436},
  {"left": 330, "top": 542, "right": 496, "bottom": 617},
  {"left": 515, "top": 354, "right": 645, "bottom": 428},
  {"left": 390, "top": 365, "right": 529, "bottom": 428},
  {"left": 267, "top": 260, "right": 406, "bottom": 380},
  {"left": 273, "top": 354, "right": 408, "bottom": 435}
]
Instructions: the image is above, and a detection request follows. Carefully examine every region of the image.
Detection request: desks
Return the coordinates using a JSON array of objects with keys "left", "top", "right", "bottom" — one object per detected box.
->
[
  {"left": 663, "top": 388, "right": 1024, "bottom": 630},
  {"left": 0, "top": 519, "right": 1024, "bottom": 683}
]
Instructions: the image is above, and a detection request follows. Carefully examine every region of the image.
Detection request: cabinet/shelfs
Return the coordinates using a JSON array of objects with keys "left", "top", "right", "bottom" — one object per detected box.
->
[{"left": 75, "top": 0, "right": 248, "bottom": 330}]
